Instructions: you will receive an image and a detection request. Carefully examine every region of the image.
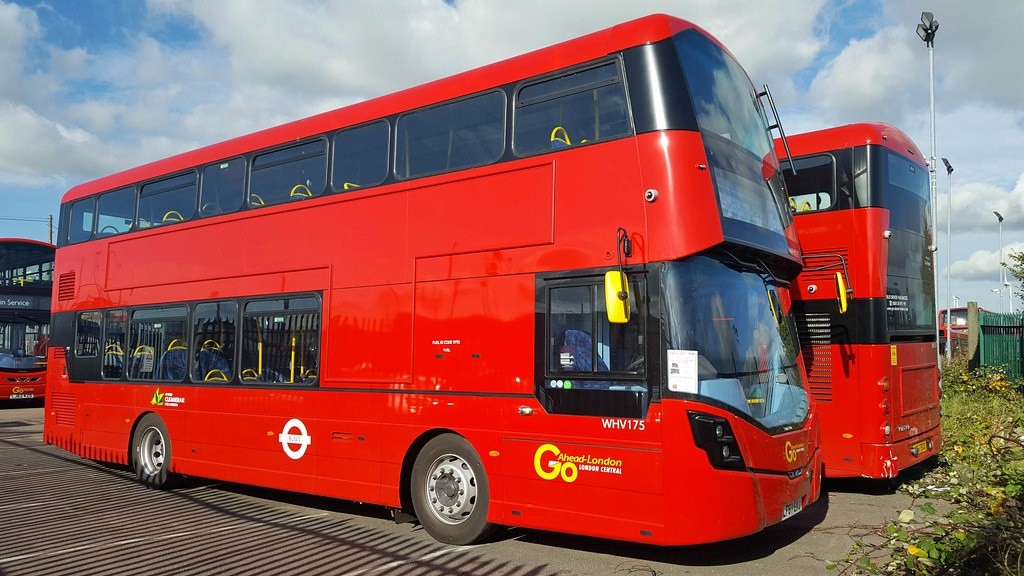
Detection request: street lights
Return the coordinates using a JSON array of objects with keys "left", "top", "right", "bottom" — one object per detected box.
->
[
  {"left": 916, "top": 11, "right": 943, "bottom": 404},
  {"left": 993, "top": 211, "right": 1004, "bottom": 312},
  {"left": 940, "top": 156, "right": 954, "bottom": 366}
]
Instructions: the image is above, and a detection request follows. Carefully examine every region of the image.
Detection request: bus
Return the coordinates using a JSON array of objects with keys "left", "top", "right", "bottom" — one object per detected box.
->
[
  {"left": 42, "top": 13, "right": 853, "bottom": 548},
  {"left": 1, "top": 236, "right": 57, "bottom": 404},
  {"left": 765, "top": 122, "right": 944, "bottom": 479},
  {"left": 937, "top": 306, "right": 1020, "bottom": 348}
]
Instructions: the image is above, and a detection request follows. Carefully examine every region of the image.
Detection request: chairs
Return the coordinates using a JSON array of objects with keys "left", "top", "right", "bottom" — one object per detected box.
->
[
  {"left": 104, "top": 340, "right": 318, "bottom": 385},
  {"left": 96, "top": 181, "right": 362, "bottom": 241},
  {"left": 555, "top": 329, "right": 614, "bottom": 389},
  {"left": 550, "top": 127, "right": 572, "bottom": 151}
]
